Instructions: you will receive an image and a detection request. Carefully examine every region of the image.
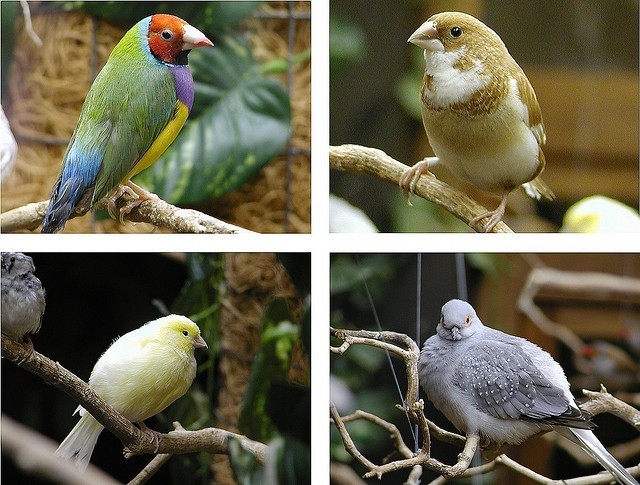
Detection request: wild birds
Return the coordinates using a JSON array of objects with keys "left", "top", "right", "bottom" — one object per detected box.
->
[
  {"left": 51, "top": 314, "right": 209, "bottom": 474},
  {"left": 1, "top": 252, "right": 47, "bottom": 368},
  {"left": 39, "top": 13, "right": 215, "bottom": 234},
  {"left": 399, "top": 12, "right": 557, "bottom": 233}
]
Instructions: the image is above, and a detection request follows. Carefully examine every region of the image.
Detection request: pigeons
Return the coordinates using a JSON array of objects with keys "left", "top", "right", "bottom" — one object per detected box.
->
[{"left": 417, "top": 298, "right": 640, "bottom": 485}]
